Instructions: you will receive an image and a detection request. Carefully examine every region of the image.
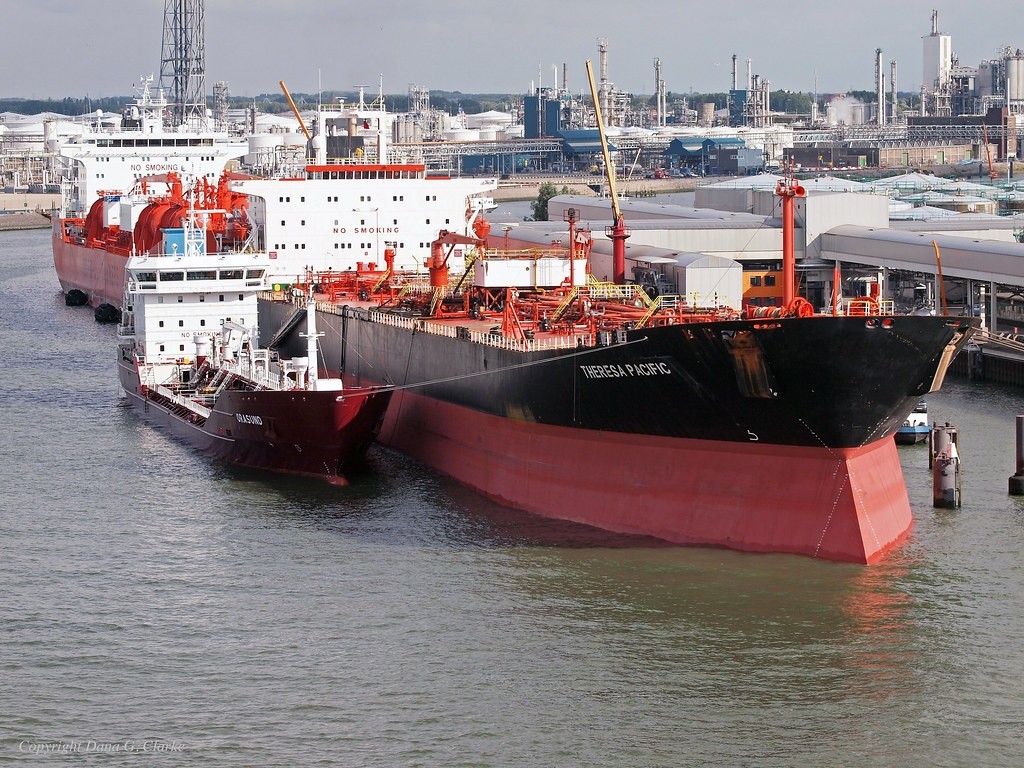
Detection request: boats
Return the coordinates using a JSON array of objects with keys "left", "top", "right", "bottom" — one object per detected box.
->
[
  {"left": 117, "top": 212, "right": 395, "bottom": 489},
  {"left": 50, "top": 214, "right": 263, "bottom": 323},
  {"left": 895, "top": 399, "right": 932, "bottom": 443},
  {"left": 243, "top": 258, "right": 978, "bottom": 567},
  {"left": 469, "top": 197, "right": 500, "bottom": 213}
]
